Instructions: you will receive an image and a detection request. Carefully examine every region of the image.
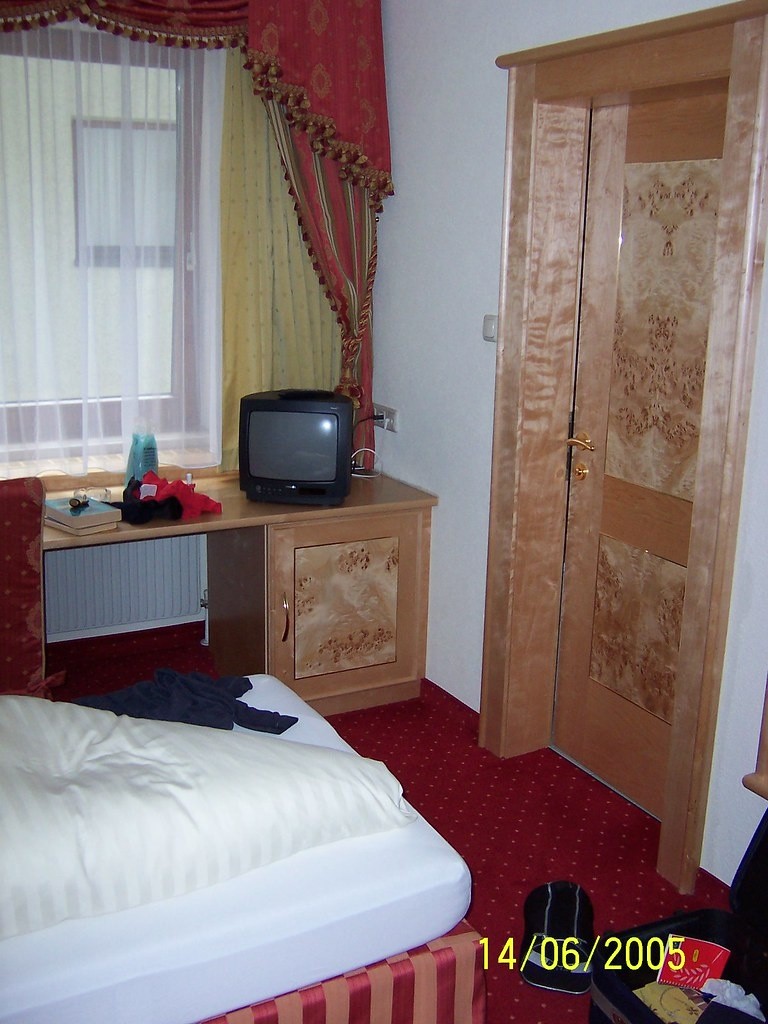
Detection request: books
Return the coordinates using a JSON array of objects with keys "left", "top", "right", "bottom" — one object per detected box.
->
[
  {"left": 44, "top": 497, "right": 123, "bottom": 536},
  {"left": 657, "top": 933, "right": 732, "bottom": 991}
]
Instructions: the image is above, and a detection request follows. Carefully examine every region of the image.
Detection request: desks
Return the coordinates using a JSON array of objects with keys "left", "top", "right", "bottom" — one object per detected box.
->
[{"left": 38, "top": 469, "right": 438, "bottom": 719}]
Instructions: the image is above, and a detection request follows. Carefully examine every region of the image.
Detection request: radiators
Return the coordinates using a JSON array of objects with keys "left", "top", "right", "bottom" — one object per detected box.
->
[{"left": 44, "top": 533, "right": 210, "bottom": 647}]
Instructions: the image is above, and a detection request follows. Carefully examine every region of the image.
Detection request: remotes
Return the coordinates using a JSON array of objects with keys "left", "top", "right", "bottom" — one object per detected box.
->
[{"left": 279, "top": 389, "right": 335, "bottom": 399}]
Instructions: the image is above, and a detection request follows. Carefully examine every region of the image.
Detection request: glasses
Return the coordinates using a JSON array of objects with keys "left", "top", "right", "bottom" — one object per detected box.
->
[{"left": 74, "top": 487, "right": 112, "bottom": 501}]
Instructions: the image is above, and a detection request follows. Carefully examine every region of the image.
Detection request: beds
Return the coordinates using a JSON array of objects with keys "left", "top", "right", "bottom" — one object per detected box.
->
[{"left": 0, "top": 674, "right": 485, "bottom": 1024}]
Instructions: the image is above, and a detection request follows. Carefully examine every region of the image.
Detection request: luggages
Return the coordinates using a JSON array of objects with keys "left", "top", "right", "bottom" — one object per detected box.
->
[{"left": 591, "top": 808, "right": 767, "bottom": 1023}]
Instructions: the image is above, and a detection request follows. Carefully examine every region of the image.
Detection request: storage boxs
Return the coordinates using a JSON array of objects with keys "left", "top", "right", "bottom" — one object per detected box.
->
[{"left": 589, "top": 807, "right": 768, "bottom": 1024}]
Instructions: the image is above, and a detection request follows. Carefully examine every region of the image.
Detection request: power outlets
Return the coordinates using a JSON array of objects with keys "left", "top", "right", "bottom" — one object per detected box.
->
[{"left": 373, "top": 403, "right": 397, "bottom": 433}]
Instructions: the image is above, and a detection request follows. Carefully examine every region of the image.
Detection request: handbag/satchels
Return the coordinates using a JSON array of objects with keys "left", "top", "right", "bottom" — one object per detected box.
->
[{"left": 520, "top": 880, "right": 594, "bottom": 994}]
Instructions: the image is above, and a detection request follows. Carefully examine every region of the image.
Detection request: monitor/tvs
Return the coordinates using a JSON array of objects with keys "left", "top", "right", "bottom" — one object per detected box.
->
[{"left": 239, "top": 389, "right": 353, "bottom": 505}]
